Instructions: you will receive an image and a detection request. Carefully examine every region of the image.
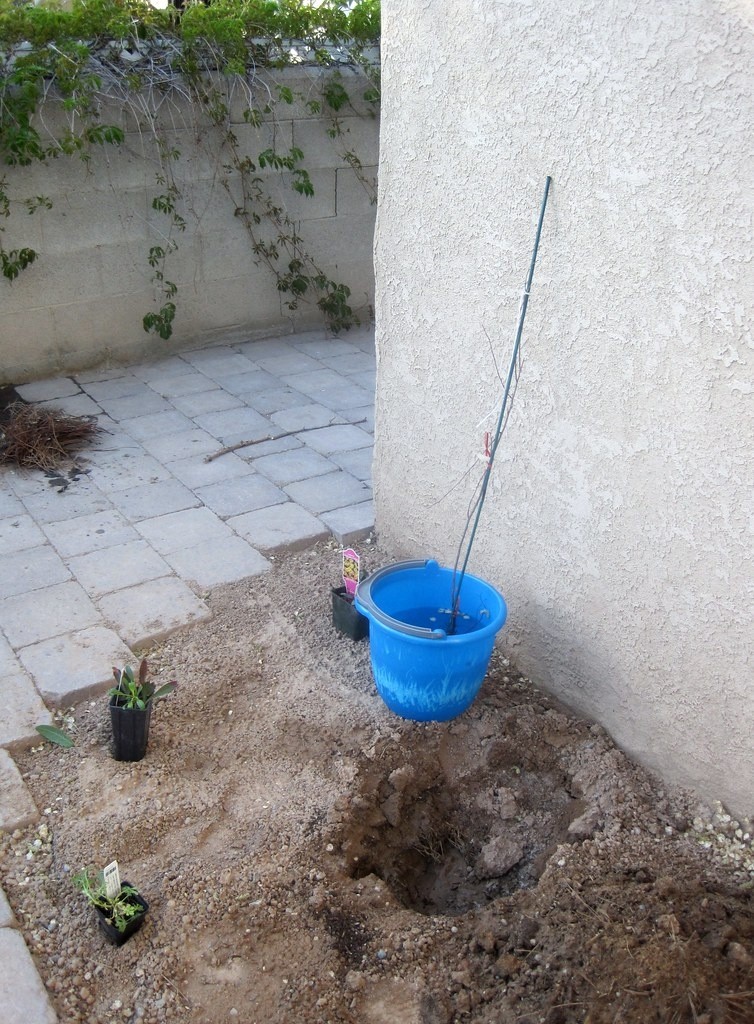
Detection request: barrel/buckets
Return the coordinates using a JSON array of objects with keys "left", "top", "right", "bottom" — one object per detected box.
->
[{"left": 353, "top": 558, "right": 508, "bottom": 722}]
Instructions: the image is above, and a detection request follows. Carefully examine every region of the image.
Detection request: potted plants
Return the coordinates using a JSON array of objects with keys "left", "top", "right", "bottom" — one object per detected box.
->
[
  {"left": 108, "top": 658, "right": 179, "bottom": 763},
  {"left": 331, "top": 587, "right": 369, "bottom": 642},
  {"left": 75, "top": 869, "right": 151, "bottom": 946}
]
